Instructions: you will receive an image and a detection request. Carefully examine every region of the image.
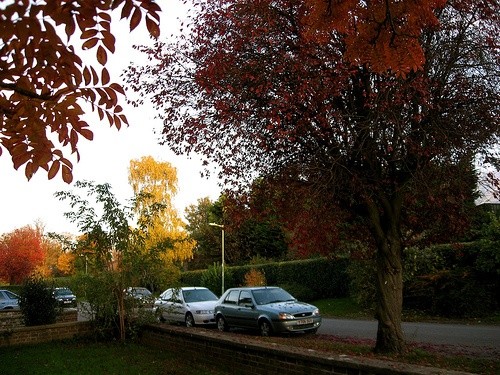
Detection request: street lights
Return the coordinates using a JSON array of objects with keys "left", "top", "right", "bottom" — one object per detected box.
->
[
  {"left": 79, "top": 253, "right": 88, "bottom": 296},
  {"left": 209, "top": 222, "right": 225, "bottom": 296}
]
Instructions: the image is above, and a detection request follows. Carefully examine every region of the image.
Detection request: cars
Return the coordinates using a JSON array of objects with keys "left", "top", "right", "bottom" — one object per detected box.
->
[
  {"left": 214, "top": 286, "right": 323, "bottom": 336},
  {"left": 153, "top": 287, "right": 221, "bottom": 328},
  {"left": 0, "top": 290, "right": 27, "bottom": 310},
  {"left": 123, "top": 287, "right": 157, "bottom": 308},
  {"left": 51, "top": 288, "right": 77, "bottom": 307}
]
